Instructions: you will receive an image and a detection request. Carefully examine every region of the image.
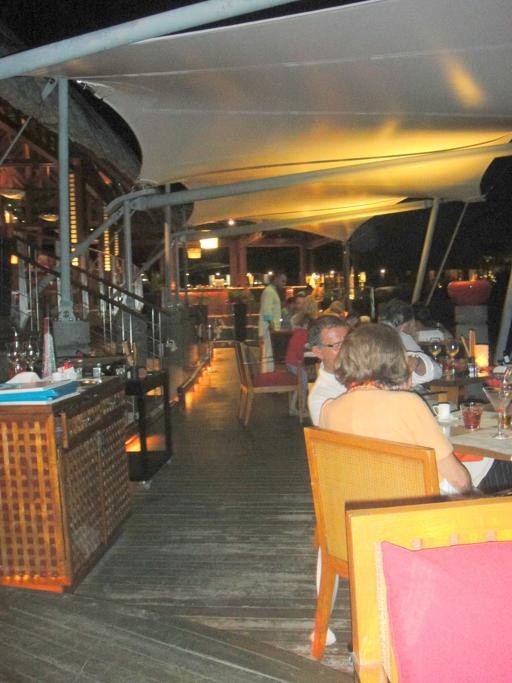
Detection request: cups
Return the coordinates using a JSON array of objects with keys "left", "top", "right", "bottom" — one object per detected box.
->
[
  {"left": 459, "top": 402, "right": 484, "bottom": 428},
  {"left": 432, "top": 403, "right": 450, "bottom": 419}
]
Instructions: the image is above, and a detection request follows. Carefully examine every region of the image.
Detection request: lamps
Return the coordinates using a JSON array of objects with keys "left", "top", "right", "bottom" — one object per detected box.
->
[{"left": 186, "top": 240, "right": 202, "bottom": 259}]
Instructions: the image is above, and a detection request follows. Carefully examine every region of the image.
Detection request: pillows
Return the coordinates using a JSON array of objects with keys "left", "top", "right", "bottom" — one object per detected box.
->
[{"left": 376, "top": 540, "right": 511, "bottom": 683}]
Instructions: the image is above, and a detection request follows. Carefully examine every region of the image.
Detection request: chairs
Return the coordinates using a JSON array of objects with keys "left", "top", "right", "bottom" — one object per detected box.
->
[
  {"left": 345, "top": 492, "right": 512, "bottom": 683},
  {"left": 302, "top": 425, "right": 442, "bottom": 662},
  {"left": 232, "top": 321, "right": 300, "bottom": 427}
]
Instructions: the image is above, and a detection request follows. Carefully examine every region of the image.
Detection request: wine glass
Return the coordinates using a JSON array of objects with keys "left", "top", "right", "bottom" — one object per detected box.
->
[
  {"left": 482, "top": 379, "right": 511, "bottom": 429},
  {"left": 429, "top": 337, "right": 442, "bottom": 362},
  {"left": 484, "top": 387, "right": 511, "bottom": 440},
  {"left": 7, "top": 341, "right": 39, "bottom": 375},
  {"left": 445, "top": 339, "right": 460, "bottom": 361}
]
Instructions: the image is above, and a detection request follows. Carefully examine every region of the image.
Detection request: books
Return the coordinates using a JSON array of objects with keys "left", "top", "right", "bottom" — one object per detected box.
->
[{"left": 0, "top": 378, "right": 73, "bottom": 394}]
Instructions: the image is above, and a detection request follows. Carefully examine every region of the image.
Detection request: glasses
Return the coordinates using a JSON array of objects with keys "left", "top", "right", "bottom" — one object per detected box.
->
[{"left": 318, "top": 340, "right": 344, "bottom": 352}]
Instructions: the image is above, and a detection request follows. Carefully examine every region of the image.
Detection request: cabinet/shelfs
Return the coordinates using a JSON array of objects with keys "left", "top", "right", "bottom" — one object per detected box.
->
[{"left": 0, "top": 368, "right": 172, "bottom": 592}]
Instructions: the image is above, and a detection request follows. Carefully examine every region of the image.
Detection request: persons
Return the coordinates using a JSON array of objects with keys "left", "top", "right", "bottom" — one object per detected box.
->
[
  {"left": 318, "top": 320, "right": 482, "bottom": 494},
  {"left": 258, "top": 269, "right": 288, "bottom": 374},
  {"left": 408, "top": 301, "right": 454, "bottom": 342},
  {"left": 375, "top": 296, "right": 425, "bottom": 353},
  {"left": 303, "top": 314, "right": 355, "bottom": 429},
  {"left": 280, "top": 287, "right": 372, "bottom": 417},
  {"left": 405, "top": 350, "right": 444, "bottom": 385}
]
